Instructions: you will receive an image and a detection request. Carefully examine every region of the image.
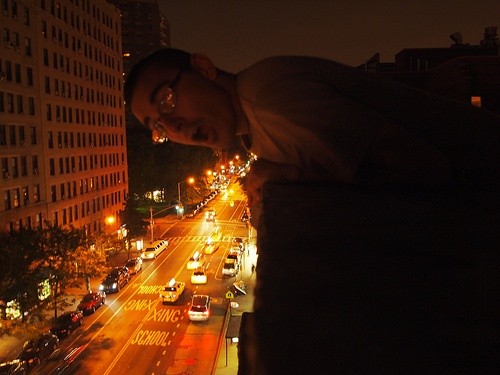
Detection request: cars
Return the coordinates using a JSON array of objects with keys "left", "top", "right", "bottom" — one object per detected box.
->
[
  {"left": 201, "top": 239, "right": 219, "bottom": 255},
  {"left": 185, "top": 155, "right": 258, "bottom": 222},
  {"left": 140, "top": 240, "right": 168, "bottom": 260},
  {"left": 191, "top": 268, "right": 207, "bottom": 285},
  {"left": 77, "top": 292, "right": 105, "bottom": 315},
  {"left": 161, "top": 278, "right": 186, "bottom": 304},
  {"left": 187, "top": 256, "right": 204, "bottom": 270},
  {"left": 125, "top": 256, "right": 142, "bottom": 274},
  {"left": 0, "top": 331, "right": 58, "bottom": 375}
]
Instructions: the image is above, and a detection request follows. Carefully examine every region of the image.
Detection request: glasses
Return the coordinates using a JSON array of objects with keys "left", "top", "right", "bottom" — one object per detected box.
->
[{"left": 151, "top": 65, "right": 191, "bottom": 143}]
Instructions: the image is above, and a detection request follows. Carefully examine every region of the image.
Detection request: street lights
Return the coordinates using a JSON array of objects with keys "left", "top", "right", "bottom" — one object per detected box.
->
[
  {"left": 84, "top": 217, "right": 116, "bottom": 293},
  {"left": 178, "top": 178, "right": 194, "bottom": 204}
]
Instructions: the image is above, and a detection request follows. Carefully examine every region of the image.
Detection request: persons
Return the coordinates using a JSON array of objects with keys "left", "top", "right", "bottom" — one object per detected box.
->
[{"left": 124, "top": 47, "right": 500, "bottom": 375}]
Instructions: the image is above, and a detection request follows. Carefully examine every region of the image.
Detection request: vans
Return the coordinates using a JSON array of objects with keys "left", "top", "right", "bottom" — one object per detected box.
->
[{"left": 222, "top": 237, "right": 244, "bottom": 277}]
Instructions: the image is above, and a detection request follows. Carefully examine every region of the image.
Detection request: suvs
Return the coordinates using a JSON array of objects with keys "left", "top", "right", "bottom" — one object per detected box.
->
[
  {"left": 48, "top": 310, "right": 84, "bottom": 341},
  {"left": 187, "top": 294, "right": 212, "bottom": 322},
  {"left": 99, "top": 266, "right": 130, "bottom": 294}
]
